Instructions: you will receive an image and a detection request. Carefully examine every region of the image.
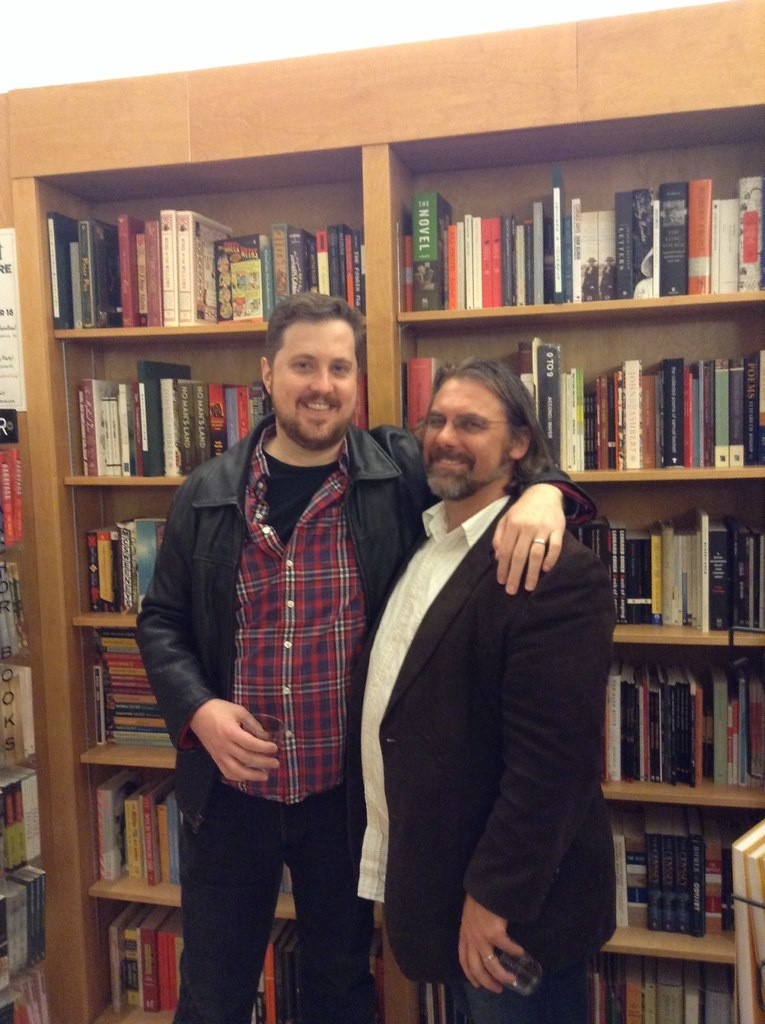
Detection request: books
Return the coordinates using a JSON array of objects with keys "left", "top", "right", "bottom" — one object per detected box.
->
[{"left": 0, "top": 171, "right": 765, "bottom": 1024}]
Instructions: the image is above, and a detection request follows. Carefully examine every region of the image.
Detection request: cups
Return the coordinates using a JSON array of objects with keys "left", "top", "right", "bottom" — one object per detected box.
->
[{"left": 493, "top": 935, "right": 543, "bottom": 998}]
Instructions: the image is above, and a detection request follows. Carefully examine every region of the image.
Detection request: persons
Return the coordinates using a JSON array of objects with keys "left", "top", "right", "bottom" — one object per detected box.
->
[
  {"left": 136, "top": 293, "right": 598, "bottom": 1024},
  {"left": 347, "top": 359, "right": 618, "bottom": 1024}
]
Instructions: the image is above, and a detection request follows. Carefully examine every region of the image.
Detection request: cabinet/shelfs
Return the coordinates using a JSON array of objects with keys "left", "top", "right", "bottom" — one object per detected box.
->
[{"left": 0, "top": 0, "right": 765, "bottom": 1024}]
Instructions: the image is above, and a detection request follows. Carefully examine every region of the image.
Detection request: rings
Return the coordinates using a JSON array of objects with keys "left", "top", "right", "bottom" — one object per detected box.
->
[
  {"left": 482, "top": 955, "right": 494, "bottom": 962},
  {"left": 534, "top": 538, "right": 546, "bottom": 545}
]
